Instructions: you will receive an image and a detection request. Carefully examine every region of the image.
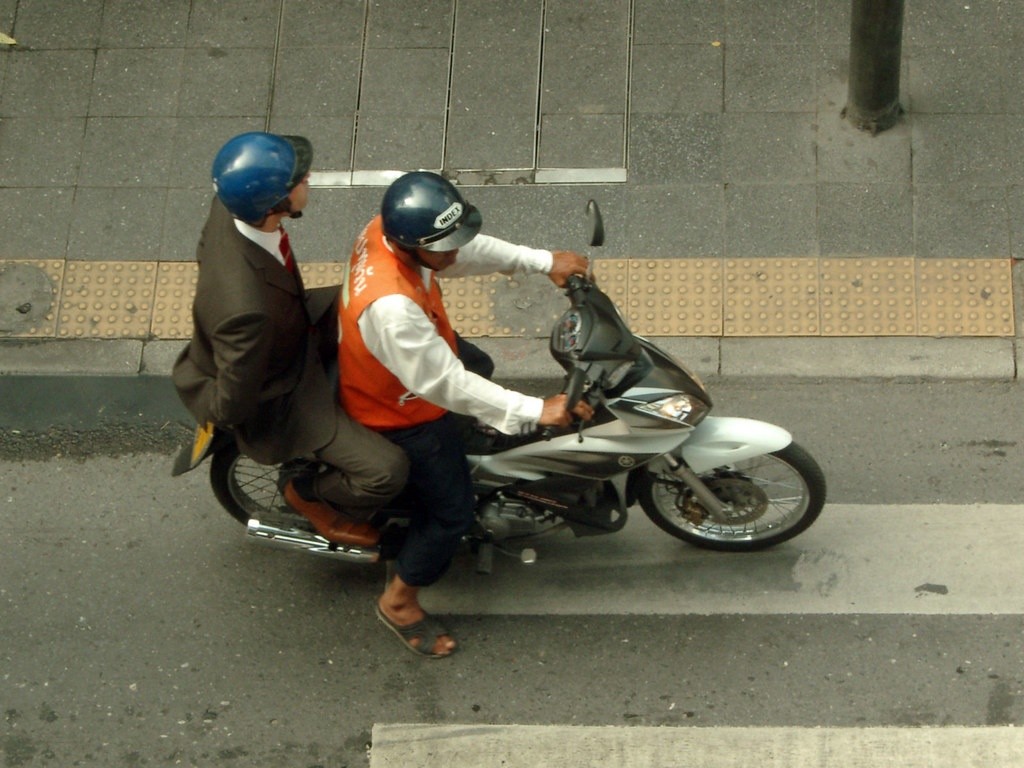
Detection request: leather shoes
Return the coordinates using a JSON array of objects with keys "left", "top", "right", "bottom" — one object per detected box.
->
[{"left": 284, "top": 474, "right": 383, "bottom": 548}]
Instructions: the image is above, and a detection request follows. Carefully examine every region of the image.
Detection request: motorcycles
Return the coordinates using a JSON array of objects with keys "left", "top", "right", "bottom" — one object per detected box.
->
[{"left": 171, "top": 199, "right": 826, "bottom": 568}]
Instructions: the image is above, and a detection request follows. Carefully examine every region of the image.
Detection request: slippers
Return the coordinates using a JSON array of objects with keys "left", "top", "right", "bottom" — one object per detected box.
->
[{"left": 372, "top": 598, "right": 458, "bottom": 660}]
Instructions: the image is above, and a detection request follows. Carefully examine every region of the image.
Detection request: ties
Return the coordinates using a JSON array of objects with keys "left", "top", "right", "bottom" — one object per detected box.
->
[{"left": 276, "top": 223, "right": 301, "bottom": 275}]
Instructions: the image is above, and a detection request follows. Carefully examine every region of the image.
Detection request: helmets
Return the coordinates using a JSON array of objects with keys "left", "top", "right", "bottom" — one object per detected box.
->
[
  {"left": 210, "top": 131, "right": 314, "bottom": 222},
  {"left": 380, "top": 171, "right": 483, "bottom": 252}
]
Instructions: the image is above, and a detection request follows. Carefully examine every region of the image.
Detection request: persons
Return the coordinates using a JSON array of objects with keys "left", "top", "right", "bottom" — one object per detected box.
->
[
  {"left": 171, "top": 132, "right": 409, "bottom": 526},
  {"left": 337, "top": 170, "right": 595, "bottom": 658}
]
